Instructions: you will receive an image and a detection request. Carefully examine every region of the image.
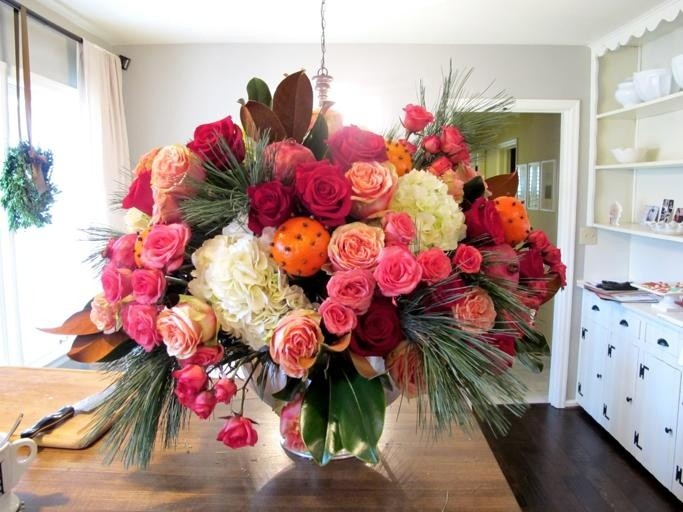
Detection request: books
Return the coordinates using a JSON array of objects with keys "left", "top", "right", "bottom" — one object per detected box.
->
[{"left": 582, "top": 279, "right": 683, "bottom": 303}]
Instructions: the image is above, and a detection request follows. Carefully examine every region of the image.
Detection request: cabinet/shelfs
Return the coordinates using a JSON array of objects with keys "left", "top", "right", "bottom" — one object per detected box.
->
[
  {"left": 586, "top": 1, "right": 682, "bottom": 244},
  {"left": 628, "top": 316, "right": 683, "bottom": 504},
  {"left": 574, "top": 289, "right": 628, "bottom": 452}
]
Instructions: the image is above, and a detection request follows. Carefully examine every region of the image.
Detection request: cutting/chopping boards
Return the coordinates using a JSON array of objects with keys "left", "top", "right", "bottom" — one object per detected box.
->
[{"left": 0, "top": 366, "right": 140, "bottom": 450}]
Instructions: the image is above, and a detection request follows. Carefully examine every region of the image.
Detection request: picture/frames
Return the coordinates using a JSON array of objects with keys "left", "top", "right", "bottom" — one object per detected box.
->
[
  {"left": 516, "top": 164, "right": 526, "bottom": 204},
  {"left": 526, "top": 162, "right": 540, "bottom": 211}
]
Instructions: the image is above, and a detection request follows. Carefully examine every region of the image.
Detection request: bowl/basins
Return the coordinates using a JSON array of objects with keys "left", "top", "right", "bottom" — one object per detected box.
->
[
  {"left": 613, "top": 147, "right": 647, "bottom": 163},
  {"left": 670, "top": 56, "right": 683, "bottom": 90},
  {"left": 633, "top": 68, "right": 680, "bottom": 101}
]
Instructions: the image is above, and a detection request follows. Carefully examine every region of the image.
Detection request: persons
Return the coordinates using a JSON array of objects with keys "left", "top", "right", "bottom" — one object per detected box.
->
[{"left": 668, "top": 200, "right": 673, "bottom": 212}]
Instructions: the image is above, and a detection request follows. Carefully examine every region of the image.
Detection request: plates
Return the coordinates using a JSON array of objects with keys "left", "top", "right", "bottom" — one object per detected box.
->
[
  {"left": 629, "top": 279, "right": 682, "bottom": 296},
  {"left": 645, "top": 221, "right": 683, "bottom": 233}
]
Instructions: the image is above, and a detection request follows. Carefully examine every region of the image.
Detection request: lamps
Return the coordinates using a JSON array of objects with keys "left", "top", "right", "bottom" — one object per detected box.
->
[{"left": 308, "top": 1, "right": 342, "bottom": 135}]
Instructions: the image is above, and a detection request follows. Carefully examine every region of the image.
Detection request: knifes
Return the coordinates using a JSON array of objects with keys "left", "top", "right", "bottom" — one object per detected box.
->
[{"left": 20, "top": 378, "right": 129, "bottom": 438}]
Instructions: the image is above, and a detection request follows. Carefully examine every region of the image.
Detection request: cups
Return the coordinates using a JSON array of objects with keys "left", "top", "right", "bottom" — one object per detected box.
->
[{"left": 0, "top": 432, "right": 37, "bottom": 512}]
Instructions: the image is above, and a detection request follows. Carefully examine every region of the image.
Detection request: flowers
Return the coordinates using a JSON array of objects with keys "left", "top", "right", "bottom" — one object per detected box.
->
[{"left": 38, "top": 70, "right": 568, "bottom": 472}]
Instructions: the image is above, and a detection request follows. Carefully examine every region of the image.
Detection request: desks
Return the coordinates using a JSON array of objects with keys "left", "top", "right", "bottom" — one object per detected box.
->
[{"left": 0, "top": 367, "right": 522, "bottom": 512}]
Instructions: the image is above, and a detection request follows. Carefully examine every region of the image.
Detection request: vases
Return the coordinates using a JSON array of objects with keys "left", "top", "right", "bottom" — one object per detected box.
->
[{"left": 239, "top": 358, "right": 359, "bottom": 461}]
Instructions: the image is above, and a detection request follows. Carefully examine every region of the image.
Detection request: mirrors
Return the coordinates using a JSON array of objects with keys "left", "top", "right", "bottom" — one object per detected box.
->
[{"left": 540, "top": 159, "right": 557, "bottom": 212}]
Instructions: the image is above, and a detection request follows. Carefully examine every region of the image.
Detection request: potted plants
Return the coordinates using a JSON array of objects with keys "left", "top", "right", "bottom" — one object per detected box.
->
[{"left": 0, "top": 139, "right": 63, "bottom": 235}]
